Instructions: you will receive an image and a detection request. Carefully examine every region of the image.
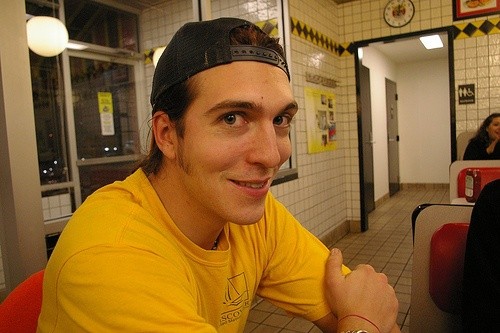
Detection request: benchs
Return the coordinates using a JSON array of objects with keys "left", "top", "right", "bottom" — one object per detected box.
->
[{"left": 410, "top": 159, "right": 500, "bottom": 333}]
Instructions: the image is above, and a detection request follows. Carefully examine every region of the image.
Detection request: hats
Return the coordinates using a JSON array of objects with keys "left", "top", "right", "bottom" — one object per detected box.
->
[{"left": 149, "top": 17, "right": 291, "bottom": 107}]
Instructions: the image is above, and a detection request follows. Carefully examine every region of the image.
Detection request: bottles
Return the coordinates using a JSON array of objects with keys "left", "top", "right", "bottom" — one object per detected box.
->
[{"left": 464, "top": 169, "right": 481, "bottom": 203}]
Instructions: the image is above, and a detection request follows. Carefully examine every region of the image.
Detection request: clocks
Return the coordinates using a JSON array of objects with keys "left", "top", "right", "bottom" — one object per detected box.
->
[{"left": 383, "top": 0, "right": 415, "bottom": 28}]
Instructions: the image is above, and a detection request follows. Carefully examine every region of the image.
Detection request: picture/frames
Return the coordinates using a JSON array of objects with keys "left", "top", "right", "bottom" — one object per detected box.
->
[{"left": 452, "top": 0, "right": 500, "bottom": 21}]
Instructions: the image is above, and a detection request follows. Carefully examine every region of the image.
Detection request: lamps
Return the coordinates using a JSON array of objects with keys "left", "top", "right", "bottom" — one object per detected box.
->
[
  {"left": 26, "top": 15, "right": 68, "bottom": 56},
  {"left": 146, "top": 47, "right": 167, "bottom": 68}
]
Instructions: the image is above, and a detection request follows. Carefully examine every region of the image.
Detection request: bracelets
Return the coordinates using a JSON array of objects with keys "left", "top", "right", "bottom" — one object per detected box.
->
[
  {"left": 337, "top": 314, "right": 380, "bottom": 332},
  {"left": 339, "top": 328, "right": 370, "bottom": 332}
]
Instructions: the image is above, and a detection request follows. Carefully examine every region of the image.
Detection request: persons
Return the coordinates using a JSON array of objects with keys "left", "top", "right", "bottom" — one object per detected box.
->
[
  {"left": 463, "top": 112, "right": 500, "bottom": 160},
  {"left": 449, "top": 176, "right": 500, "bottom": 333},
  {"left": 36, "top": 16, "right": 403, "bottom": 333}
]
